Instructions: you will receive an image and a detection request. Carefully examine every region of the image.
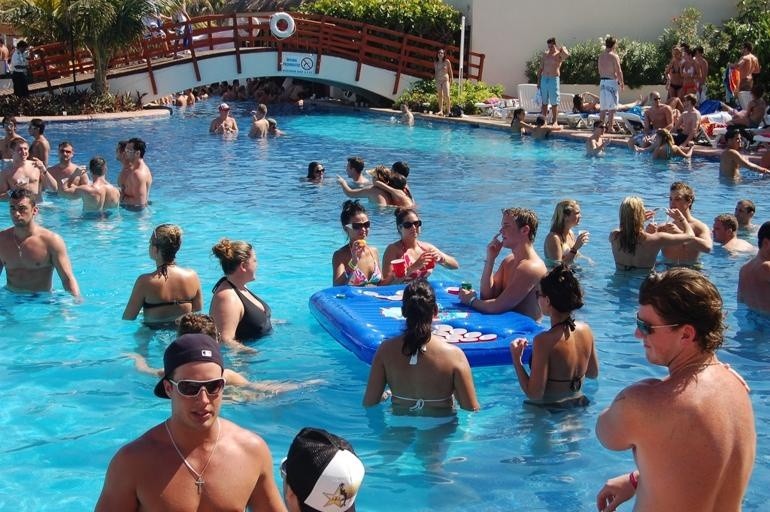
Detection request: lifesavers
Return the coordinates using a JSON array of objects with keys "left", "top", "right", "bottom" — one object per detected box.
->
[{"left": 270, "top": 12, "right": 295, "bottom": 37}]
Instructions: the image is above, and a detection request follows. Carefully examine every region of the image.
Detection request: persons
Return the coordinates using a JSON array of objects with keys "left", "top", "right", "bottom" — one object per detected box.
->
[
  {"left": 512, "top": 37, "right": 768, "bottom": 178},
  {"left": 596, "top": 265, "right": 756, "bottom": 511},
  {"left": 734, "top": 200, "right": 761, "bottom": 231},
  {"left": 208, "top": 238, "right": 271, "bottom": 343},
  {"left": 333, "top": 199, "right": 397, "bottom": 286},
  {"left": 646, "top": 181, "right": 712, "bottom": 263},
  {"left": 336, "top": 156, "right": 415, "bottom": 206},
  {"left": 544, "top": 199, "right": 592, "bottom": 261},
  {"left": 1, "top": 0, "right": 454, "bottom": 139},
  {"left": 127, "top": 316, "right": 328, "bottom": 406},
  {"left": 458, "top": 206, "right": 549, "bottom": 322},
  {"left": 121, "top": 224, "right": 203, "bottom": 322},
  {"left": 737, "top": 221, "right": 770, "bottom": 311},
  {"left": 510, "top": 264, "right": 598, "bottom": 459},
  {"left": 712, "top": 213, "right": 758, "bottom": 252},
  {"left": 608, "top": 195, "right": 695, "bottom": 271},
  {"left": 383, "top": 207, "right": 458, "bottom": 285},
  {"left": 93, "top": 334, "right": 287, "bottom": 511},
  {"left": 2, "top": 114, "right": 153, "bottom": 210},
  {"left": 0, "top": 188, "right": 81, "bottom": 296},
  {"left": 306, "top": 162, "right": 326, "bottom": 179},
  {"left": 362, "top": 280, "right": 481, "bottom": 476}
]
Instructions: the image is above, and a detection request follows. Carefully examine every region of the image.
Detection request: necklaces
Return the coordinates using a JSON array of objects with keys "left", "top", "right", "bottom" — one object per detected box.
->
[
  {"left": 161, "top": 417, "right": 221, "bottom": 493},
  {"left": 13, "top": 226, "right": 36, "bottom": 257},
  {"left": 669, "top": 359, "right": 722, "bottom": 377}
]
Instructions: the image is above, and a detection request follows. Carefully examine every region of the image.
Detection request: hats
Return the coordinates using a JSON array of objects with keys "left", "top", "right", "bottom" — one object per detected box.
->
[
  {"left": 155, "top": 333, "right": 224, "bottom": 399},
  {"left": 218, "top": 103, "right": 231, "bottom": 111},
  {"left": 365, "top": 168, "right": 389, "bottom": 184}
]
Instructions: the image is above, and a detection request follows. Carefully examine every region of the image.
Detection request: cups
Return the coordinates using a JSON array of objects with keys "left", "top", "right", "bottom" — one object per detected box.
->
[
  {"left": 425, "top": 254, "right": 435, "bottom": 269},
  {"left": 579, "top": 230, "right": 588, "bottom": 245},
  {"left": 61, "top": 178, "right": 70, "bottom": 189},
  {"left": 432, "top": 324, "right": 498, "bottom": 343},
  {"left": 390, "top": 258, "right": 404, "bottom": 277},
  {"left": 18, "top": 177, "right": 29, "bottom": 190}
]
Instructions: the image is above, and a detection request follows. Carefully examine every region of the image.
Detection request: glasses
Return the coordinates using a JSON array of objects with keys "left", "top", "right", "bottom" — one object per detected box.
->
[
  {"left": 344, "top": 219, "right": 371, "bottom": 230},
  {"left": 399, "top": 219, "right": 422, "bottom": 229},
  {"left": 4, "top": 122, "right": 15, "bottom": 128},
  {"left": 279, "top": 456, "right": 289, "bottom": 479},
  {"left": 634, "top": 308, "right": 684, "bottom": 337},
  {"left": 534, "top": 289, "right": 545, "bottom": 300},
  {"left": 116, "top": 147, "right": 135, "bottom": 154},
  {"left": 652, "top": 207, "right": 670, "bottom": 227},
  {"left": 653, "top": 97, "right": 661, "bottom": 100},
  {"left": 59, "top": 149, "right": 72, "bottom": 154},
  {"left": 315, "top": 168, "right": 326, "bottom": 174},
  {"left": 167, "top": 377, "right": 226, "bottom": 398}
]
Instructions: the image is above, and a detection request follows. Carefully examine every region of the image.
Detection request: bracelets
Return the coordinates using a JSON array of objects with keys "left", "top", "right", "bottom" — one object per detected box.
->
[
  {"left": 436, "top": 253, "right": 449, "bottom": 266},
  {"left": 344, "top": 258, "right": 358, "bottom": 279},
  {"left": 629, "top": 471, "right": 639, "bottom": 490}
]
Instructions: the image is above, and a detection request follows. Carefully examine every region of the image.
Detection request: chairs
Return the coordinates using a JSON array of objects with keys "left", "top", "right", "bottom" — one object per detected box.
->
[{"left": 471, "top": 82, "right": 770, "bottom": 148}]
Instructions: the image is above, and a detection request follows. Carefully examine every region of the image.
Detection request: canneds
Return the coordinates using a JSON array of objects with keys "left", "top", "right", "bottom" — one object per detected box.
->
[{"left": 462, "top": 283, "right": 472, "bottom": 291}]
